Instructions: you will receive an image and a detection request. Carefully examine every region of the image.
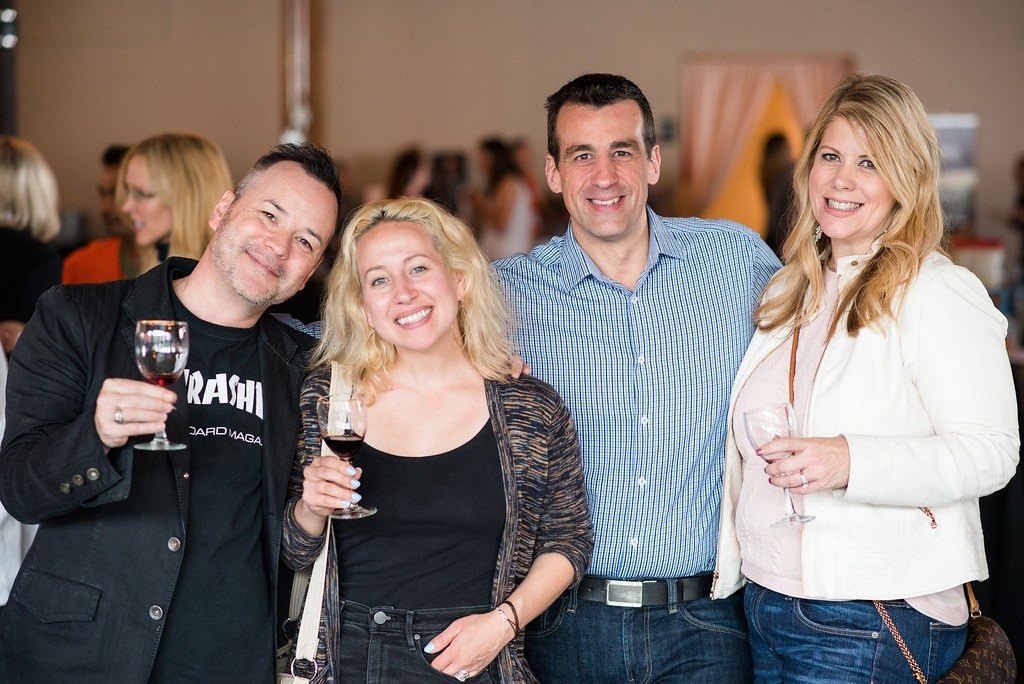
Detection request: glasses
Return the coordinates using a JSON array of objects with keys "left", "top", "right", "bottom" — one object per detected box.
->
[{"left": 122, "top": 183, "right": 153, "bottom": 200}]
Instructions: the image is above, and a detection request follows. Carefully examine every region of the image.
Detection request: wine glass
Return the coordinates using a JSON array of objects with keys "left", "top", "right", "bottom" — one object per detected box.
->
[
  {"left": 133, "top": 319, "right": 189, "bottom": 451},
  {"left": 742, "top": 402, "right": 817, "bottom": 529},
  {"left": 316, "top": 391, "right": 378, "bottom": 518}
]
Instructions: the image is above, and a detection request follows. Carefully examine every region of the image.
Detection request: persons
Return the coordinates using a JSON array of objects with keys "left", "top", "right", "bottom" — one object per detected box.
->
[
  {"left": 0, "top": 142, "right": 533, "bottom": 684},
  {"left": 279, "top": 194, "right": 597, "bottom": 684},
  {"left": 710, "top": 73, "right": 1020, "bottom": 684},
  {"left": 758, "top": 129, "right": 796, "bottom": 263},
  {"left": 1, "top": 132, "right": 548, "bottom": 684},
  {"left": 268, "top": 70, "right": 783, "bottom": 684}
]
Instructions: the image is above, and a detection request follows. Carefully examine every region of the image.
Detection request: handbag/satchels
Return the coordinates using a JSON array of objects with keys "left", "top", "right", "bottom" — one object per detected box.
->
[{"left": 924, "top": 616, "right": 1017, "bottom": 684}]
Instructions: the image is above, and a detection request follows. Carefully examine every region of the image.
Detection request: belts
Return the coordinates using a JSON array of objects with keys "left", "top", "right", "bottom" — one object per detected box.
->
[{"left": 562, "top": 577, "right": 712, "bottom": 607}]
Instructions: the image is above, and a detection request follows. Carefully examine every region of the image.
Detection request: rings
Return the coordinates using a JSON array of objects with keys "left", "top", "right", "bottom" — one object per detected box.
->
[
  {"left": 454, "top": 669, "right": 469, "bottom": 682},
  {"left": 114, "top": 408, "right": 123, "bottom": 424},
  {"left": 801, "top": 475, "right": 808, "bottom": 489}
]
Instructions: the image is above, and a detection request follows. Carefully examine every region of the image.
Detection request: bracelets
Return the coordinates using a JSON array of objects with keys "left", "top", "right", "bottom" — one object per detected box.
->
[{"left": 495, "top": 600, "right": 521, "bottom": 643}]
